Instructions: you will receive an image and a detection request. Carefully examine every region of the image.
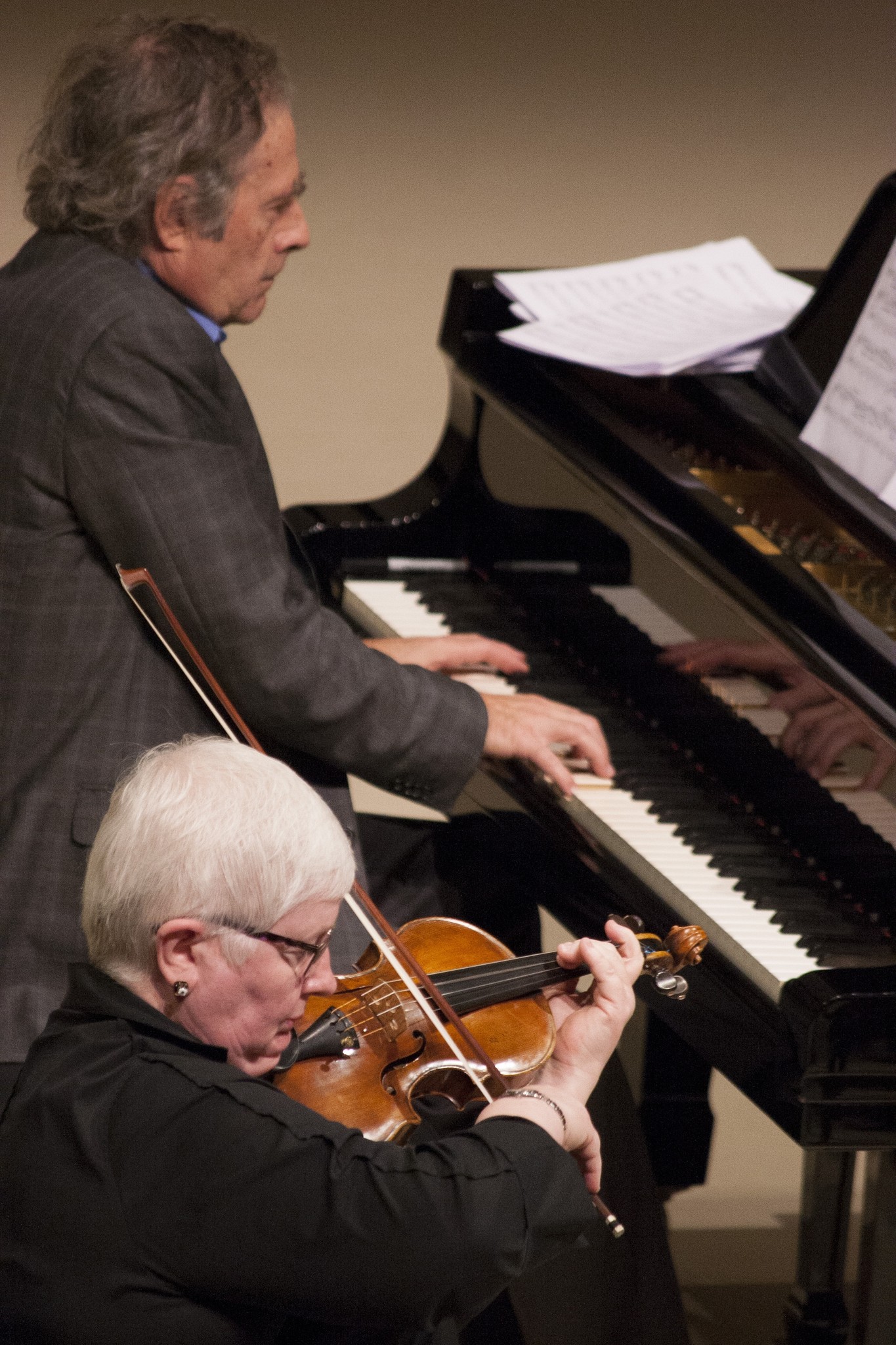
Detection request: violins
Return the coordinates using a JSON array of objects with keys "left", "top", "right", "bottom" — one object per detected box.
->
[{"left": 264, "top": 912, "right": 710, "bottom": 1155}]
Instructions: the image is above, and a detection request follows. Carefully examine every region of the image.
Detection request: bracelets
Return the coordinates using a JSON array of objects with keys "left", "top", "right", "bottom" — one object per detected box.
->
[{"left": 495, "top": 1088, "right": 567, "bottom": 1146}]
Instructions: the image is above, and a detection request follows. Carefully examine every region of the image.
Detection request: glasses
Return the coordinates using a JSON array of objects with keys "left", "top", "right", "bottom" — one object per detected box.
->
[{"left": 152, "top": 913, "right": 332, "bottom": 981}]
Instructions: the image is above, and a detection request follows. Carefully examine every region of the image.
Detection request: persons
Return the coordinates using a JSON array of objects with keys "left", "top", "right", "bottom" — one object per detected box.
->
[
  {"left": 0, "top": 734, "right": 647, "bottom": 1345},
  {"left": 625, "top": 626, "right": 895, "bottom": 1199},
  {"left": 0, "top": 10, "right": 633, "bottom": 1075}
]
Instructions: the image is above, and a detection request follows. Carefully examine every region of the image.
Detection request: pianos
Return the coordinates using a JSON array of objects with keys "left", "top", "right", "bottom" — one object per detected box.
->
[{"left": 276, "top": 168, "right": 896, "bottom": 1345}]
